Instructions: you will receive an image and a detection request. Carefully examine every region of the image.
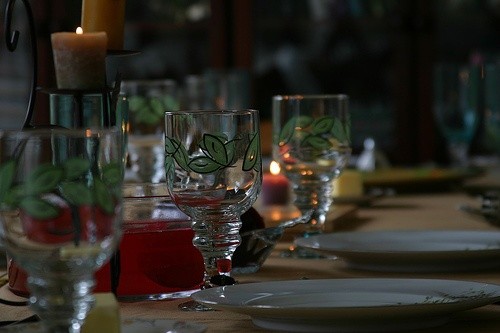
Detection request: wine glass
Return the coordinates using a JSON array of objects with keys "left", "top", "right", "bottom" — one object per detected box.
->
[
  {"left": 164, "top": 110, "right": 264, "bottom": 313},
  {"left": 271, "top": 95, "right": 353, "bottom": 263},
  {"left": 0, "top": 126, "right": 127, "bottom": 333},
  {"left": 113, "top": 78, "right": 179, "bottom": 198},
  {"left": 436, "top": 63, "right": 500, "bottom": 180}
]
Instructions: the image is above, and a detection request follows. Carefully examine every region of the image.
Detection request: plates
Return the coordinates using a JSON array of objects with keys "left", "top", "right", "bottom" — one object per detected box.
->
[
  {"left": 190, "top": 279, "right": 499, "bottom": 332},
  {"left": 291, "top": 230, "right": 499, "bottom": 276}
]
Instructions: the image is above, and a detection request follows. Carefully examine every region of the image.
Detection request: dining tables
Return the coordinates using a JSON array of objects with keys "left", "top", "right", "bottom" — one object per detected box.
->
[{"left": 1, "top": 191, "right": 500, "bottom": 332}]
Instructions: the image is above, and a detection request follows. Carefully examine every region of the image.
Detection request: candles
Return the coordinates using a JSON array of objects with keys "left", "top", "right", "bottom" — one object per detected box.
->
[
  {"left": 51, "top": 26, "right": 108, "bottom": 90},
  {"left": 260, "top": 160, "right": 289, "bottom": 204}
]
